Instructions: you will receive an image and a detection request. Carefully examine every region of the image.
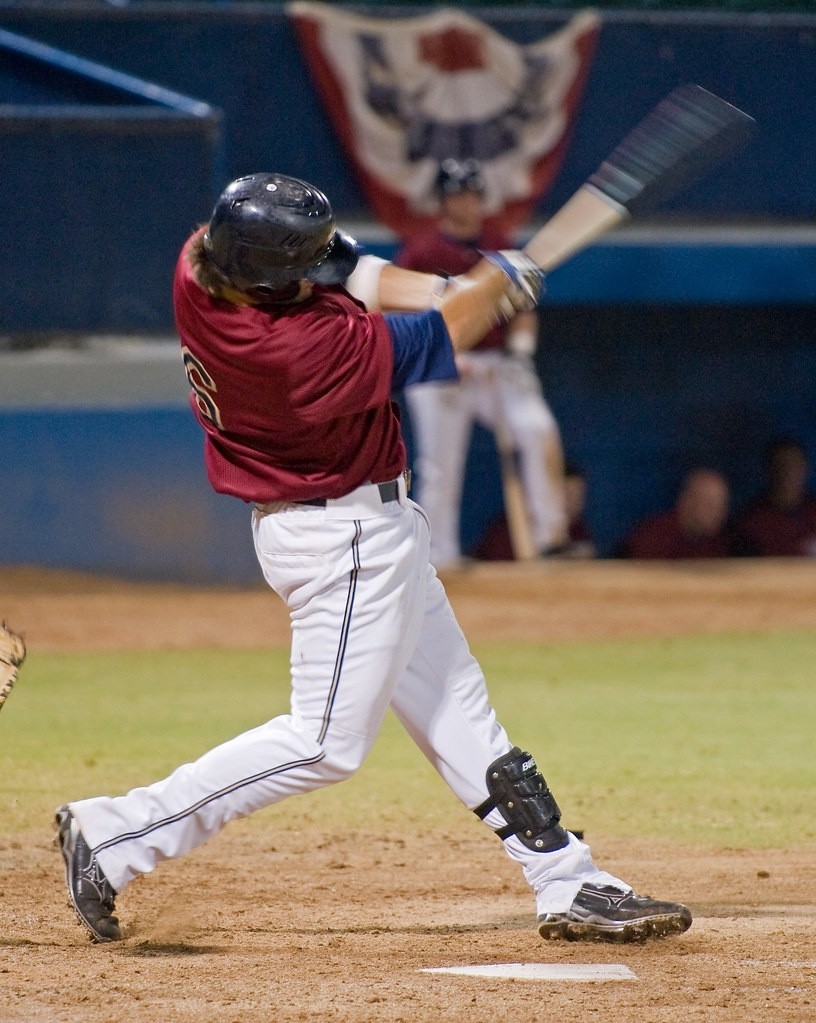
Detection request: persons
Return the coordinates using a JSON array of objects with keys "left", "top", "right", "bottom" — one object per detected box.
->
[
  {"left": 51, "top": 170, "right": 696, "bottom": 945},
  {"left": 384, "top": 152, "right": 595, "bottom": 568},
  {"left": 477, "top": 454, "right": 592, "bottom": 561},
  {"left": 615, "top": 464, "right": 755, "bottom": 560},
  {"left": 727, "top": 437, "right": 816, "bottom": 558}
]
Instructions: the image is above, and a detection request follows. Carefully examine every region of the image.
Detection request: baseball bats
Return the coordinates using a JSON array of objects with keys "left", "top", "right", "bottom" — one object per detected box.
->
[{"left": 481, "top": 83, "right": 754, "bottom": 317}]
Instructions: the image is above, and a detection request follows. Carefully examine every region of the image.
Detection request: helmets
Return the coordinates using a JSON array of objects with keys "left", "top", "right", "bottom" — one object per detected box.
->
[{"left": 203, "top": 172, "right": 361, "bottom": 291}]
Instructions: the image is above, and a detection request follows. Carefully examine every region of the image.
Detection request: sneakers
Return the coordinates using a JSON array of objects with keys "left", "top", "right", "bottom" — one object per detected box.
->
[
  {"left": 55, "top": 802, "right": 123, "bottom": 943},
  {"left": 537, "top": 882, "right": 693, "bottom": 943}
]
